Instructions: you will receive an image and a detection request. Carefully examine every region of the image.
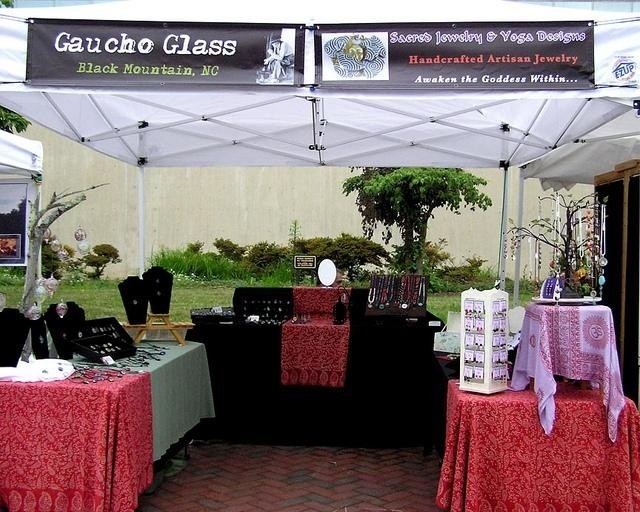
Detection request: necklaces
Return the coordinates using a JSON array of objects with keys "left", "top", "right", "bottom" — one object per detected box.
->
[
  {"left": 367, "top": 272, "right": 429, "bottom": 312},
  {"left": 531, "top": 193, "right": 609, "bottom": 306}
]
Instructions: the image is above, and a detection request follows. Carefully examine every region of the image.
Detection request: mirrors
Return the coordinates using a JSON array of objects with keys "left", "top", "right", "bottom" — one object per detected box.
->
[{"left": 318, "top": 258, "right": 337, "bottom": 286}]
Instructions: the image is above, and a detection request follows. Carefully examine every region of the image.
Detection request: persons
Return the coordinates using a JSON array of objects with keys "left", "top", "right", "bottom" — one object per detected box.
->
[{"left": 262, "top": 33, "right": 294, "bottom": 85}]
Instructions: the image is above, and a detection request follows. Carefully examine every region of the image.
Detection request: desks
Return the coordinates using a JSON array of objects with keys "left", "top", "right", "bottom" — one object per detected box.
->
[
  {"left": 60, "top": 340, "right": 216, "bottom": 493},
  {"left": 436, "top": 379, "right": 639, "bottom": 512},
  {"left": 185, "top": 307, "right": 446, "bottom": 446},
  {"left": 2, "top": 371, "right": 153, "bottom": 512}
]
